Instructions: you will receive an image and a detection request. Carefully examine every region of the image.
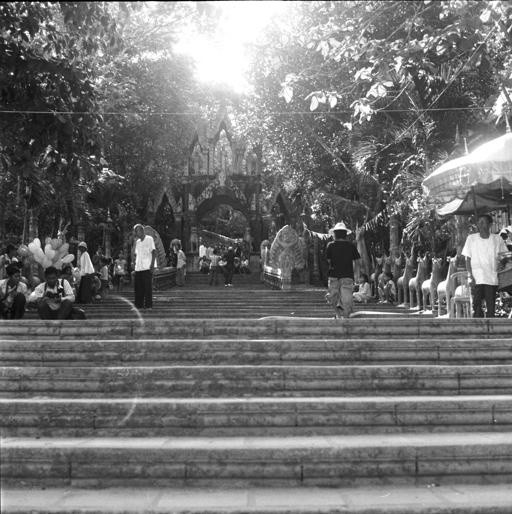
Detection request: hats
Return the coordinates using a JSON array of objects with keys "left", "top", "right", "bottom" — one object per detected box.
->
[
  {"left": 328, "top": 221, "right": 353, "bottom": 234},
  {"left": 77, "top": 241, "right": 89, "bottom": 250}
]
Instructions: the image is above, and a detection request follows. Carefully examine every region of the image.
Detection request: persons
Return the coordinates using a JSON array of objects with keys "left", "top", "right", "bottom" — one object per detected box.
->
[
  {"left": 0, "top": 263, "right": 28, "bottom": 320},
  {"left": 353, "top": 273, "right": 372, "bottom": 304},
  {"left": 377, "top": 272, "right": 396, "bottom": 305},
  {"left": 24, "top": 265, "right": 88, "bottom": 320},
  {"left": 496, "top": 215, "right": 512, "bottom": 302},
  {"left": 131, "top": 223, "right": 159, "bottom": 312},
  {"left": 18, "top": 235, "right": 250, "bottom": 305},
  {"left": 323, "top": 221, "right": 362, "bottom": 320},
  {"left": 460, "top": 214, "right": 510, "bottom": 318},
  {"left": 0, "top": 242, "right": 19, "bottom": 280}
]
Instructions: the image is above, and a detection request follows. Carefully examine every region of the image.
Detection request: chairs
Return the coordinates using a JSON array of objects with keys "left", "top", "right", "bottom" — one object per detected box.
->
[{"left": 450, "top": 271, "right": 470, "bottom": 317}]
